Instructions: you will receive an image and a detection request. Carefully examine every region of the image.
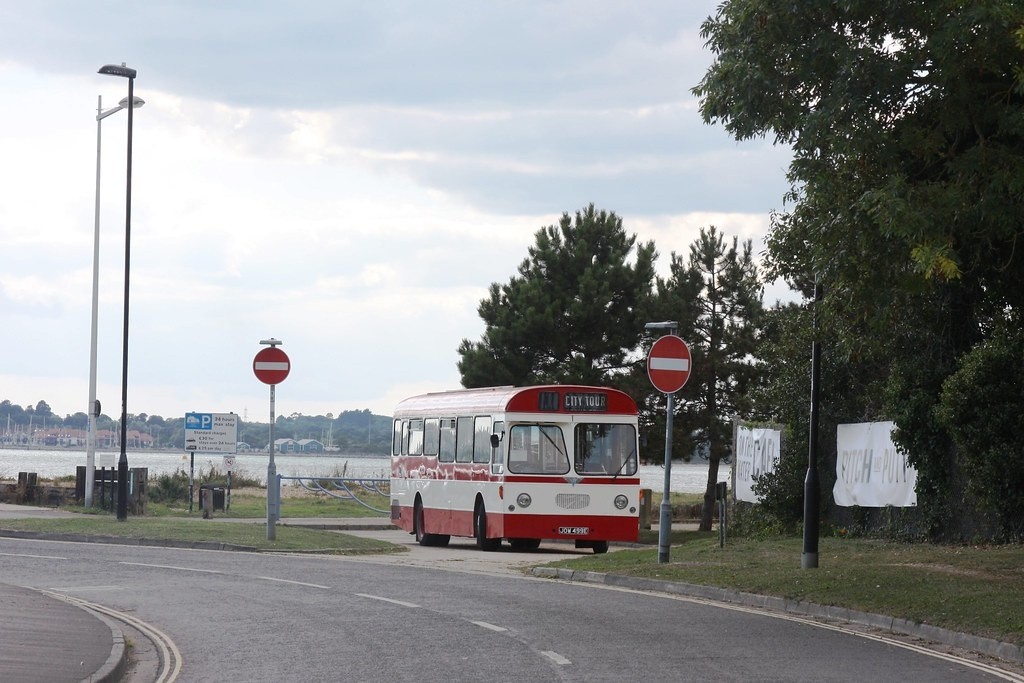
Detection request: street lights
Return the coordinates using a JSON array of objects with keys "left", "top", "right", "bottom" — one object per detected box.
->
[
  {"left": 645, "top": 321, "right": 680, "bottom": 564},
  {"left": 260, "top": 338, "right": 282, "bottom": 542},
  {"left": 83, "top": 95, "right": 146, "bottom": 510},
  {"left": 101, "top": 64, "right": 135, "bottom": 522}
]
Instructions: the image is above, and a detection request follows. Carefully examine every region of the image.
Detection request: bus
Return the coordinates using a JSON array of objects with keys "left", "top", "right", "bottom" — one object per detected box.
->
[{"left": 390, "top": 383, "right": 647, "bottom": 553}]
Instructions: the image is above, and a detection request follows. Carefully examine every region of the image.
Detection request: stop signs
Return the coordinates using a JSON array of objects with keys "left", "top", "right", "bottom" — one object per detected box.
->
[
  {"left": 252, "top": 348, "right": 290, "bottom": 384},
  {"left": 646, "top": 336, "right": 693, "bottom": 394}
]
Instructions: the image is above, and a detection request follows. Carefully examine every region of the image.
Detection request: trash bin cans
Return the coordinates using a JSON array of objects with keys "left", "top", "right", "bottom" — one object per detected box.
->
[{"left": 199, "top": 484, "right": 225, "bottom": 513}]
